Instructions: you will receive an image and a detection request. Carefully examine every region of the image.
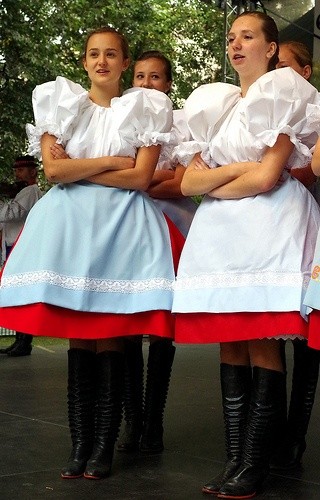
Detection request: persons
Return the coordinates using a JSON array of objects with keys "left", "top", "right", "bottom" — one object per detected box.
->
[
  {"left": 0, "top": 25, "right": 176, "bottom": 480},
  {"left": 0, "top": 155, "right": 43, "bottom": 356},
  {"left": 171, "top": 11, "right": 320, "bottom": 500},
  {"left": 112, "top": 50, "right": 198, "bottom": 472}
]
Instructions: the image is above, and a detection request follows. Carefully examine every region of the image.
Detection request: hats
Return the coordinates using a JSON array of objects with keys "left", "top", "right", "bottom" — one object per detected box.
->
[{"left": 10, "top": 156, "right": 39, "bottom": 168}]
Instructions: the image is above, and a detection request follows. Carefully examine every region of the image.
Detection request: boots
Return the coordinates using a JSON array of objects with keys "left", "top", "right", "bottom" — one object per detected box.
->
[
  {"left": 262, "top": 339, "right": 320, "bottom": 469},
  {"left": 0, "top": 332, "right": 33, "bottom": 355},
  {"left": 202, "top": 363, "right": 288, "bottom": 498},
  {"left": 61, "top": 349, "right": 125, "bottom": 478},
  {"left": 118, "top": 343, "right": 175, "bottom": 454}
]
